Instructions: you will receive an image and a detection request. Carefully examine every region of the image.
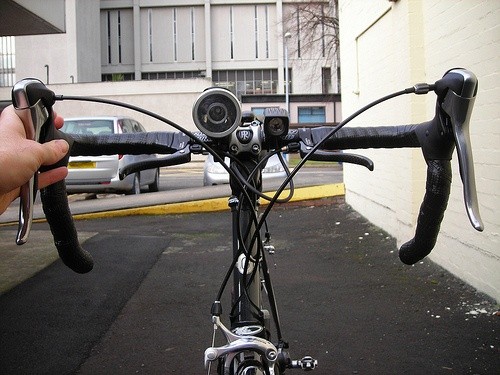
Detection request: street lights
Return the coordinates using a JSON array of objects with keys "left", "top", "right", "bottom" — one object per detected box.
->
[{"left": 284, "top": 32, "right": 292, "bottom": 115}]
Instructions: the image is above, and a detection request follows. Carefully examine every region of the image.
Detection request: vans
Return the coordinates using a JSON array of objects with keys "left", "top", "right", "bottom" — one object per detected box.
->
[{"left": 56, "top": 116, "right": 161, "bottom": 196}]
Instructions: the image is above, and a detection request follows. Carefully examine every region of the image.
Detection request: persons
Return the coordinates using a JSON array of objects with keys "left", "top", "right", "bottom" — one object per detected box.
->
[{"left": 1, "top": 98, "right": 69, "bottom": 221}]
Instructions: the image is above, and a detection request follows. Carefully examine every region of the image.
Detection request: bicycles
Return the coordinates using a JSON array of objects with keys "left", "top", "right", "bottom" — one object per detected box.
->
[{"left": 12, "top": 67, "right": 485, "bottom": 375}]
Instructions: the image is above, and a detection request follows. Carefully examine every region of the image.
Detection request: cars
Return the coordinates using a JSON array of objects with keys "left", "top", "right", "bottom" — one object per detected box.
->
[{"left": 202, "top": 145, "right": 294, "bottom": 187}]
